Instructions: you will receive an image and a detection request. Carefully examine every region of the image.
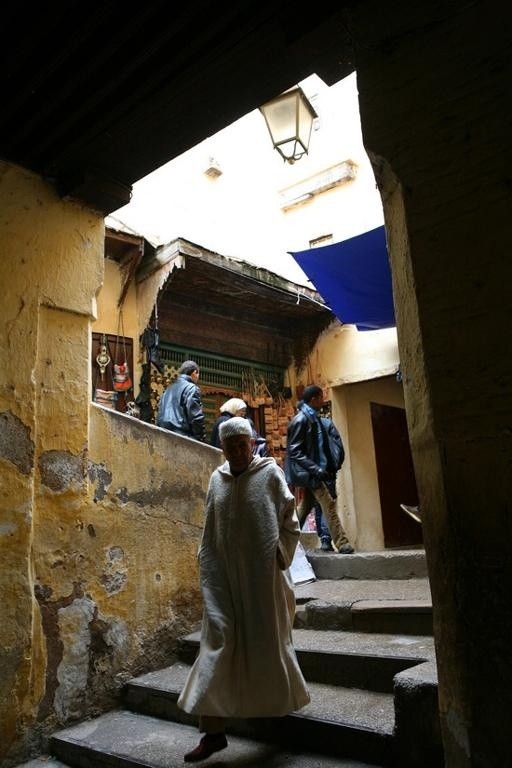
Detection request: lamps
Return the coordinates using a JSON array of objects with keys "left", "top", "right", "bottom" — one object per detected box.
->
[{"left": 258, "top": 87, "right": 318, "bottom": 165}]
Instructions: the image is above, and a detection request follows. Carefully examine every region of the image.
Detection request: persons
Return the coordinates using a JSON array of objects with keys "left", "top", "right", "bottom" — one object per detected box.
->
[
  {"left": 283, "top": 385, "right": 356, "bottom": 556},
  {"left": 155, "top": 359, "right": 208, "bottom": 444},
  {"left": 293, "top": 400, "right": 345, "bottom": 552},
  {"left": 211, "top": 395, "right": 272, "bottom": 461},
  {"left": 173, "top": 413, "right": 311, "bottom": 763}
]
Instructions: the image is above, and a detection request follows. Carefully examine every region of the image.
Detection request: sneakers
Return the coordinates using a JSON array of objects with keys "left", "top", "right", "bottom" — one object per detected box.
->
[{"left": 399, "top": 504, "right": 422, "bottom": 524}]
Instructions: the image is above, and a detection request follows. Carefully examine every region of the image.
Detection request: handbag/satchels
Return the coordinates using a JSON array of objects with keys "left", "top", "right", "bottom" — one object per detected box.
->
[
  {"left": 321, "top": 387, "right": 329, "bottom": 401},
  {"left": 112, "top": 302, "right": 132, "bottom": 393},
  {"left": 94, "top": 367, "right": 118, "bottom": 411},
  {"left": 239, "top": 372, "right": 304, "bottom": 408}
]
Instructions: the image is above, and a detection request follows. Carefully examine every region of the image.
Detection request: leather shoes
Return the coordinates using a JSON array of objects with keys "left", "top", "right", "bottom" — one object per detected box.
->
[
  {"left": 183, "top": 734, "right": 228, "bottom": 763},
  {"left": 320, "top": 538, "right": 334, "bottom": 551},
  {"left": 339, "top": 543, "right": 355, "bottom": 554}
]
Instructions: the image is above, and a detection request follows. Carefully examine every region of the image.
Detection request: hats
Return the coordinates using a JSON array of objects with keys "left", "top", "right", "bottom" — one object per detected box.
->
[{"left": 218, "top": 416, "right": 252, "bottom": 440}]
